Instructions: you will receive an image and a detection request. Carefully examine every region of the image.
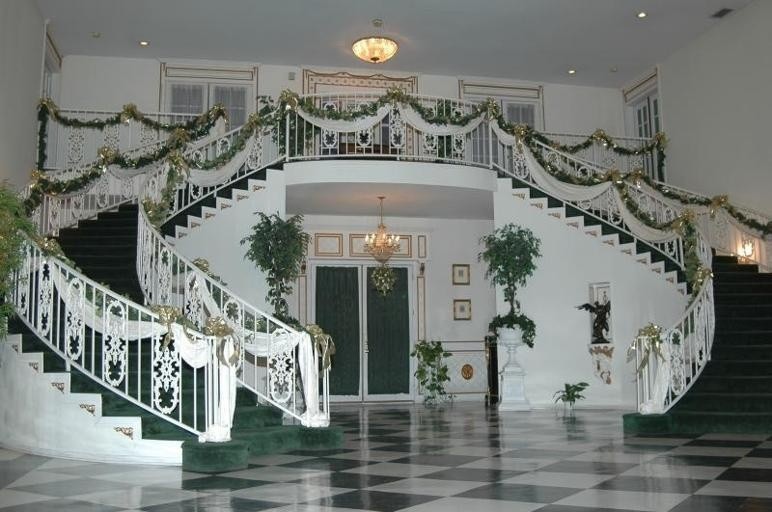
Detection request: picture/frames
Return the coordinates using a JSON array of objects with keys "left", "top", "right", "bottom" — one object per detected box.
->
[
  {"left": 452, "top": 264, "right": 470, "bottom": 285},
  {"left": 453, "top": 299, "right": 472, "bottom": 320}
]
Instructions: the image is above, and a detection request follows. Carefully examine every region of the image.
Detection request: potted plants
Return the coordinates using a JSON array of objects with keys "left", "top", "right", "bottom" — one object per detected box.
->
[
  {"left": 409, "top": 339, "right": 456, "bottom": 402},
  {"left": 552, "top": 382, "right": 590, "bottom": 409},
  {"left": 476, "top": 221, "right": 536, "bottom": 411}
]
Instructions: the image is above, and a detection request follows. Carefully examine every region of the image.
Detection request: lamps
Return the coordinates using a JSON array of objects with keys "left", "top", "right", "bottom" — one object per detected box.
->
[
  {"left": 352, "top": 19, "right": 398, "bottom": 62},
  {"left": 365, "top": 196, "right": 400, "bottom": 295},
  {"left": 743, "top": 239, "right": 753, "bottom": 257}
]
